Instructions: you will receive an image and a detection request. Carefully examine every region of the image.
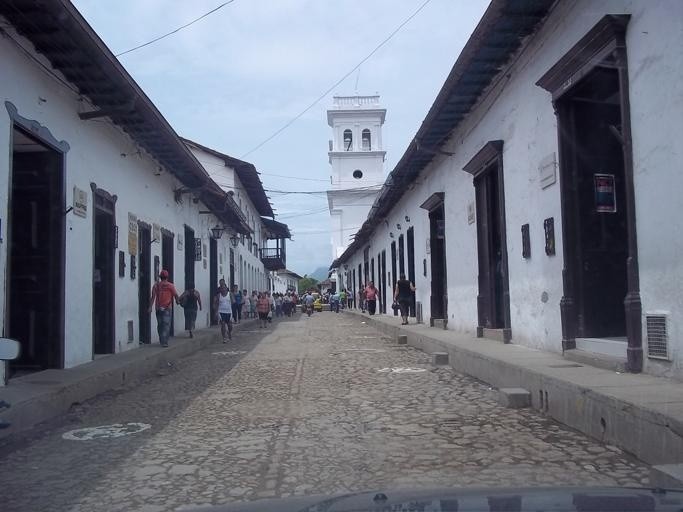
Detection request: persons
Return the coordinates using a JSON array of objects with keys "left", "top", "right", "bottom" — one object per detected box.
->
[
  {"left": 175, "top": 278, "right": 203, "bottom": 339},
  {"left": 145, "top": 269, "right": 179, "bottom": 348},
  {"left": 212, "top": 272, "right": 418, "bottom": 344}
]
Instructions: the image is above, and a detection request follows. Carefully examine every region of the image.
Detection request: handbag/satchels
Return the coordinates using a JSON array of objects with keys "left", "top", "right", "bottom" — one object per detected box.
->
[
  {"left": 392, "top": 302, "right": 400, "bottom": 309},
  {"left": 267, "top": 308, "right": 273, "bottom": 319},
  {"left": 158, "top": 305, "right": 167, "bottom": 312}
]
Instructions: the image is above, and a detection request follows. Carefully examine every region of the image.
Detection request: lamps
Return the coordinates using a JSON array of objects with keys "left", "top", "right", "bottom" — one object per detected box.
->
[
  {"left": 210, "top": 216, "right": 224, "bottom": 240},
  {"left": 229, "top": 233, "right": 240, "bottom": 247}
]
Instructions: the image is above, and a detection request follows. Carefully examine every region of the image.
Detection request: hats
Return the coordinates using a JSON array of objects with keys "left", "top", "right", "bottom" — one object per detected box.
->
[{"left": 159, "top": 271, "right": 169, "bottom": 277}]
